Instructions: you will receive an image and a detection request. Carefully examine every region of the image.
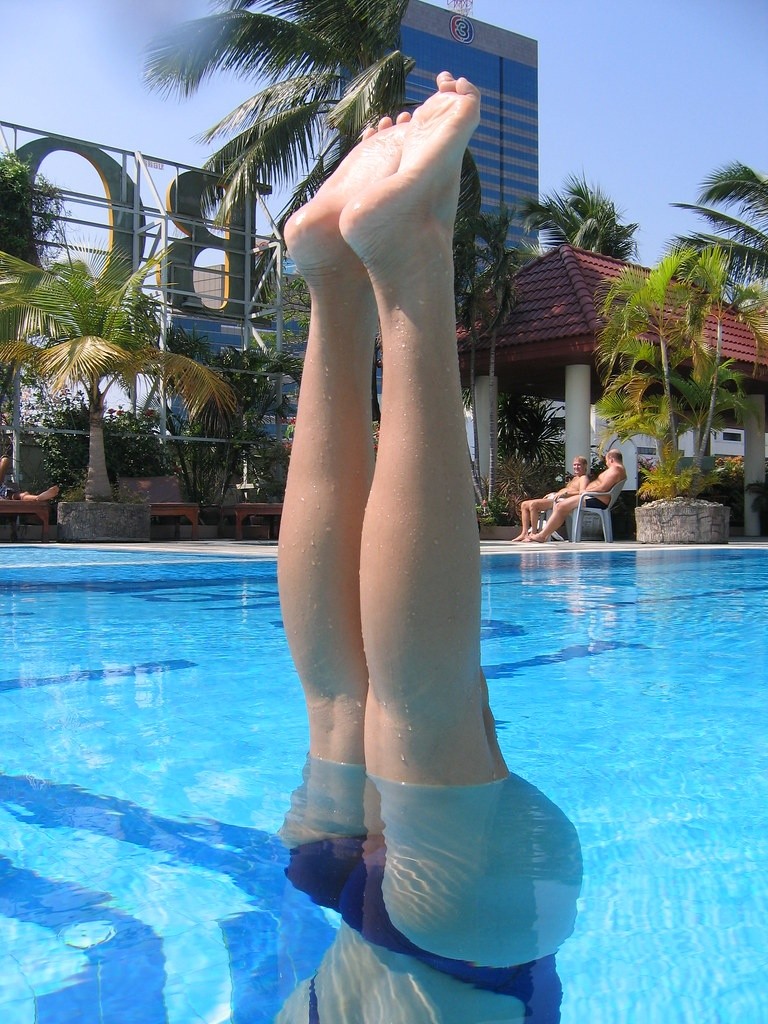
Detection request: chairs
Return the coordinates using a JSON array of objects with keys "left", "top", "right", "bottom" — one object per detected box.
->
[
  {"left": 538, "top": 489, "right": 581, "bottom": 542},
  {"left": 571, "top": 478, "right": 628, "bottom": 543}
]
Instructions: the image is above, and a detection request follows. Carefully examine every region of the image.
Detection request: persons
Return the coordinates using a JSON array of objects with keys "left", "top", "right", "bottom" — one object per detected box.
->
[
  {"left": 524, "top": 448, "right": 626, "bottom": 543},
  {"left": 274, "top": 72, "right": 583, "bottom": 786},
  {"left": 0, "top": 455, "right": 59, "bottom": 502},
  {"left": 512, "top": 456, "right": 591, "bottom": 541}
]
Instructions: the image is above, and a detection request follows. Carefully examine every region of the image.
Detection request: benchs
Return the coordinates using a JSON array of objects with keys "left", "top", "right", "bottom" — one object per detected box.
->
[
  {"left": 117, "top": 476, "right": 201, "bottom": 540},
  {"left": 0, "top": 482, "right": 50, "bottom": 543},
  {"left": 219, "top": 503, "right": 283, "bottom": 541}
]
